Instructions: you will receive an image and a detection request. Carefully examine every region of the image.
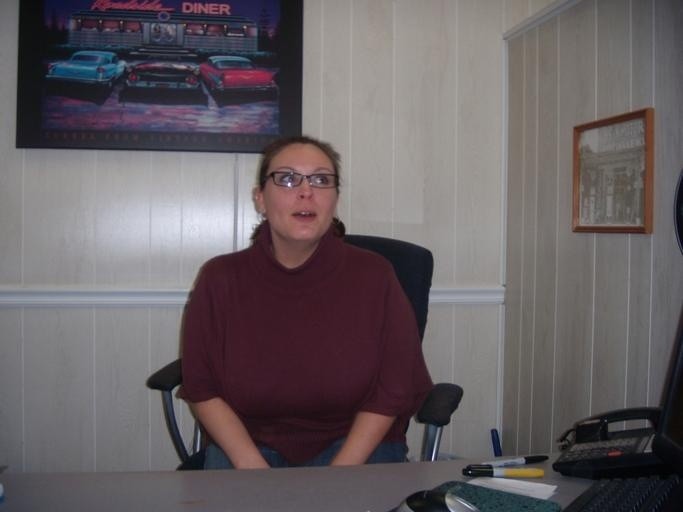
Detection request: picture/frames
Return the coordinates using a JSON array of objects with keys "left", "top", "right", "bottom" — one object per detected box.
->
[
  {"left": 572, "top": 106, "right": 655, "bottom": 235},
  {"left": 15, "top": 0, "right": 304, "bottom": 154}
]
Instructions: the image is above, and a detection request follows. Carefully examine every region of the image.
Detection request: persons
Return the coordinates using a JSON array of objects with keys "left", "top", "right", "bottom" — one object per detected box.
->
[{"left": 175, "top": 133, "right": 438, "bottom": 472}]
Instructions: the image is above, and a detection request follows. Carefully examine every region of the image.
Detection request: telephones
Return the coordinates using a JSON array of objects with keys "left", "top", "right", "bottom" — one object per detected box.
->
[{"left": 552, "top": 406, "right": 674, "bottom": 477}]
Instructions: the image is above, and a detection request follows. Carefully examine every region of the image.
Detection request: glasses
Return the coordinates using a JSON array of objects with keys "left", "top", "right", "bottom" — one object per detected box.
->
[{"left": 261, "top": 171, "right": 339, "bottom": 188}]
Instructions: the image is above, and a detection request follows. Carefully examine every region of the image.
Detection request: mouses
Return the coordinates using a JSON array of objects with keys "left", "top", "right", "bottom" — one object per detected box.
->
[{"left": 396, "top": 491, "right": 480, "bottom": 512}]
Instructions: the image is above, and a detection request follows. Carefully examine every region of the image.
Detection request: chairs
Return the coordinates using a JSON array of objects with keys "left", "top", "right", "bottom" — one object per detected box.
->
[{"left": 146, "top": 233, "right": 464, "bottom": 471}]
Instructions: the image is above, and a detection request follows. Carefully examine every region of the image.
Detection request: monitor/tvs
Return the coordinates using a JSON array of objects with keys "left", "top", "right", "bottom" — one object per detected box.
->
[{"left": 651, "top": 311, "right": 683, "bottom": 473}]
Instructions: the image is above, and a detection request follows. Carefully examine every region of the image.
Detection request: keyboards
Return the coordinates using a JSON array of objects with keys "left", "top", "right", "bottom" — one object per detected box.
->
[{"left": 562, "top": 474, "right": 683, "bottom": 512}]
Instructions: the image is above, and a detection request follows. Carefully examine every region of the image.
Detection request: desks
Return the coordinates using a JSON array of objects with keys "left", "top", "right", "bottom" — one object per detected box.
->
[{"left": 0, "top": 453, "right": 595, "bottom": 512}]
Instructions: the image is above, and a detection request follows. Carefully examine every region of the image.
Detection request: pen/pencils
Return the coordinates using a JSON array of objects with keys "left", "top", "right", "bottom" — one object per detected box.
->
[{"left": 463, "top": 456, "right": 548, "bottom": 478}]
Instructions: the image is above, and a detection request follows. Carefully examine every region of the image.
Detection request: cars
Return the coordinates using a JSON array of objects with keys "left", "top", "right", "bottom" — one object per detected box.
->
[{"left": 49, "top": 46, "right": 278, "bottom": 106}]
